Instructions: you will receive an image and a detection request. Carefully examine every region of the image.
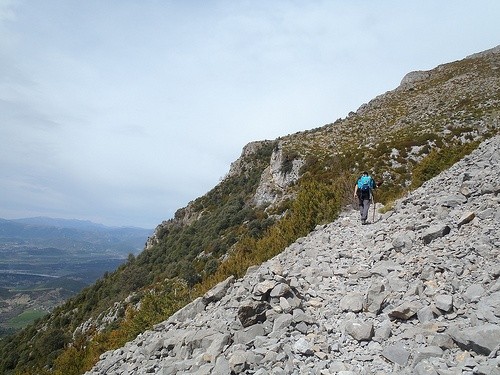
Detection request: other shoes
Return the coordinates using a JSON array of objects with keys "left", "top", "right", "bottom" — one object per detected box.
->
[{"left": 362, "top": 220, "right": 371, "bottom": 225}]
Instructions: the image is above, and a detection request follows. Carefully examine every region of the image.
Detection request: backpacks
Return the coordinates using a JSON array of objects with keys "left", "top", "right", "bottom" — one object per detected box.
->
[{"left": 359, "top": 176, "right": 377, "bottom": 190}]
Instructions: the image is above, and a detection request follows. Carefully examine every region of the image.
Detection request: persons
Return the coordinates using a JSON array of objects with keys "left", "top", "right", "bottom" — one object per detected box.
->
[{"left": 355, "top": 171, "right": 378, "bottom": 225}]
